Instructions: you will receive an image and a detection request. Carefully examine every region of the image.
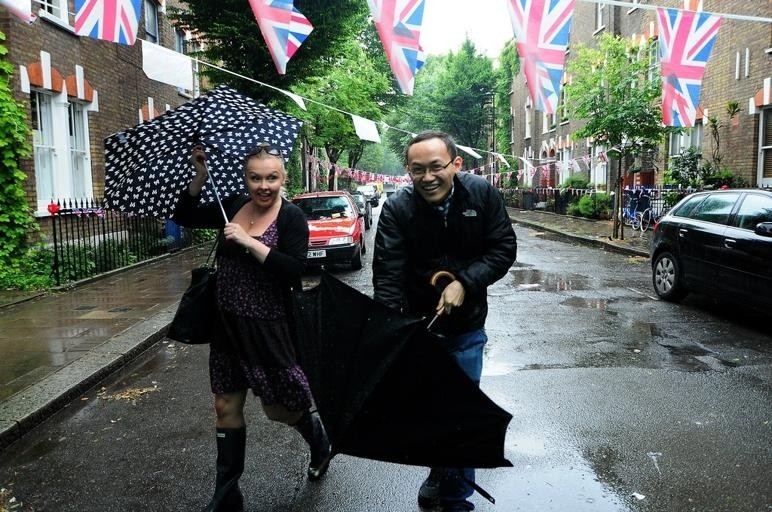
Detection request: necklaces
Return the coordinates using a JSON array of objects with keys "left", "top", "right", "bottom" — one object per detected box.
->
[{"left": 242, "top": 207, "right": 267, "bottom": 229}]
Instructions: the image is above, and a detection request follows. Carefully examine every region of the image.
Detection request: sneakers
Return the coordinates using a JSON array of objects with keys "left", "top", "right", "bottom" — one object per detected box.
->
[{"left": 418, "top": 467, "right": 475, "bottom": 511}]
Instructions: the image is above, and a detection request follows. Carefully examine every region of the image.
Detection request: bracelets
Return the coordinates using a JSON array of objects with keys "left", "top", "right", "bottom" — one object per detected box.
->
[{"left": 245, "top": 235, "right": 252, "bottom": 255}]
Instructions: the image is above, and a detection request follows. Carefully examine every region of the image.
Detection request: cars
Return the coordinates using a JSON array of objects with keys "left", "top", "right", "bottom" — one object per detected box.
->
[{"left": 290, "top": 182, "right": 383, "bottom": 269}]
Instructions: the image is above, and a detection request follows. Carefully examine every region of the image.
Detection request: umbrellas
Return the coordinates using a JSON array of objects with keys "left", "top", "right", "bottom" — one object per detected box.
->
[
  {"left": 101, "top": 81, "right": 304, "bottom": 232},
  {"left": 280, "top": 268, "right": 514, "bottom": 474}
]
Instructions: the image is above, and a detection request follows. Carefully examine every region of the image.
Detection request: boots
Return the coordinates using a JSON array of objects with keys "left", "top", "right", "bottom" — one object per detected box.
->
[
  {"left": 288, "top": 405, "right": 330, "bottom": 482},
  {"left": 201, "top": 425, "right": 246, "bottom": 512}
]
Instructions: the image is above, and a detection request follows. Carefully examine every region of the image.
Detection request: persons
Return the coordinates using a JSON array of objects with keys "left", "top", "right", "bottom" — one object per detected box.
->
[
  {"left": 173, "top": 139, "right": 344, "bottom": 508},
  {"left": 373, "top": 130, "right": 518, "bottom": 508}
]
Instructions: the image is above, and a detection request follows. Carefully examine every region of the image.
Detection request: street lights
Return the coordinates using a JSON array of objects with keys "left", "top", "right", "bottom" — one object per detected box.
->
[{"left": 485, "top": 92, "right": 495, "bottom": 188}]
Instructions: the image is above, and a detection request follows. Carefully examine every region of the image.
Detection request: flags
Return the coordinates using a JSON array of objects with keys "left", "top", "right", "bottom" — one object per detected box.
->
[
  {"left": 249, "top": 0, "right": 314, "bottom": 76},
  {"left": 71, "top": 0, "right": 142, "bottom": 46},
  {"left": 140, "top": 40, "right": 193, "bottom": 91},
  {"left": 263, "top": 81, "right": 627, "bottom": 197},
  {"left": 507, "top": 0, "right": 576, "bottom": 114},
  {"left": 362, "top": 0, "right": 426, "bottom": 100},
  {"left": 655, "top": 9, "right": 725, "bottom": 130}
]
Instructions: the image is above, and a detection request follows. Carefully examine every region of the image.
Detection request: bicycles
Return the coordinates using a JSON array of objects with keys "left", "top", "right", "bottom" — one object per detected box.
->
[{"left": 623, "top": 185, "right": 652, "bottom": 231}]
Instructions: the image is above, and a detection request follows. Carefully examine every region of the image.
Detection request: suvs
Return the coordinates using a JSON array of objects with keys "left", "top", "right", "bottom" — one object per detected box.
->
[{"left": 652, "top": 184, "right": 770, "bottom": 299}]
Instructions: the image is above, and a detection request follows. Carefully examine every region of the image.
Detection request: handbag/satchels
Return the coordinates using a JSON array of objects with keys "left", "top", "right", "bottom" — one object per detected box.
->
[{"left": 166, "top": 267, "right": 217, "bottom": 344}]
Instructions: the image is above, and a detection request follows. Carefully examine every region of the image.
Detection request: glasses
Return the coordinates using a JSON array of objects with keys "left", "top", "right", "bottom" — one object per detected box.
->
[
  {"left": 408, "top": 159, "right": 453, "bottom": 176},
  {"left": 247, "top": 145, "right": 281, "bottom": 156}
]
